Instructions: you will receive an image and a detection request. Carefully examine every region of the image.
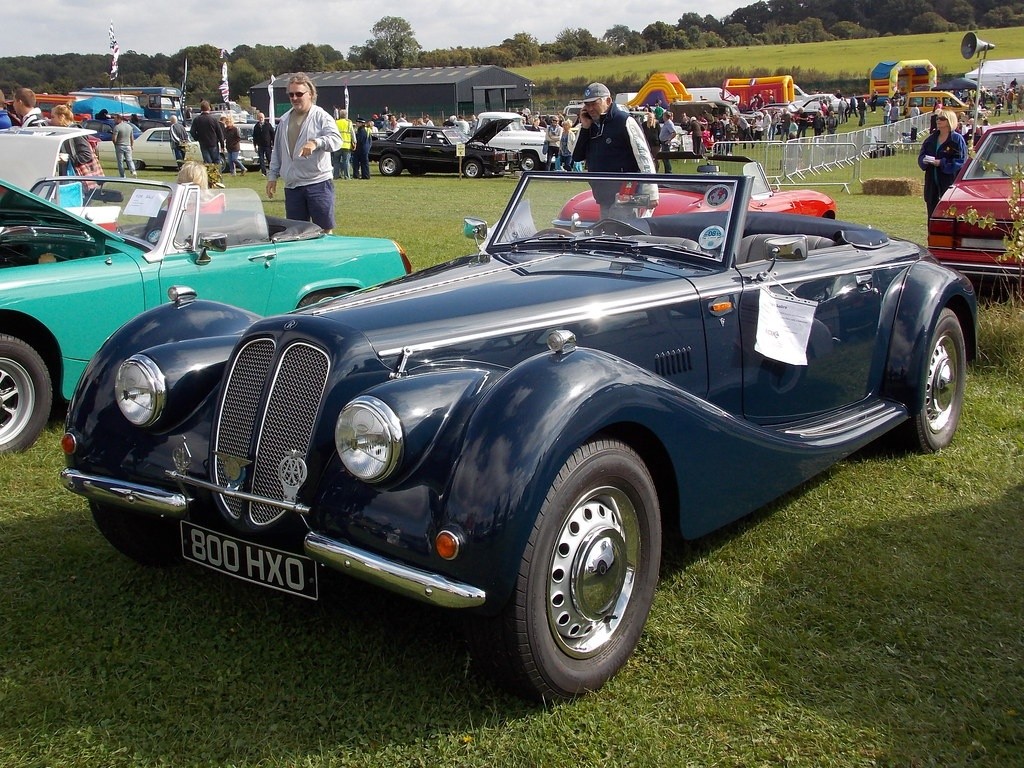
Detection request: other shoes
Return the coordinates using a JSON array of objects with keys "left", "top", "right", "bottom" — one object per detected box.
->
[
  {"left": 752, "top": 142, "right": 755, "bottom": 149},
  {"left": 262, "top": 174, "right": 265, "bottom": 177},
  {"left": 123, "top": 174, "right": 126, "bottom": 178},
  {"left": 217, "top": 184, "right": 225, "bottom": 189},
  {"left": 131, "top": 172, "right": 137, "bottom": 178},
  {"left": 240, "top": 168, "right": 247, "bottom": 176}
]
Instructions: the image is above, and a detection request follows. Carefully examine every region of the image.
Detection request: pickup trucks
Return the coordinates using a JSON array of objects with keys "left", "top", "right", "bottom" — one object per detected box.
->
[{"left": 426, "top": 110, "right": 551, "bottom": 173}]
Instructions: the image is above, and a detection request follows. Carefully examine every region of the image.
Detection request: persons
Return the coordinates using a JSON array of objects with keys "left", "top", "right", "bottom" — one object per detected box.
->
[
  {"left": 169, "top": 101, "right": 248, "bottom": 177},
  {"left": 332, "top": 105, "right": 477, "bottom": 180},
  {"left": 573, "top": 83, "right": 660, "bottom": 219},
  {"left": 95, "top": 109, "right": 139, "bottom": 178},
  {"left": 517, "top": 105, "right": 575, "bottom": 172},
  {"left": 266, "top": 76, "right": 343, "bottom": 234},
  {"left": 629, "top": 89, "right": 941, "bottom": 175},
  {"left": 956, "top": 78, "right": 1024, "bottom": 142},
  {"left": 162, "top": 161, "right": 212, "bottom": 212},
  {"left": 253, "top": 113, "right": 275, "bottom": 176},
  {"left": 918, "top": 110, "right": 969, "bottom": 214},
  {"left": 0, "top": 89, "right": 104, "bottom": 191}
]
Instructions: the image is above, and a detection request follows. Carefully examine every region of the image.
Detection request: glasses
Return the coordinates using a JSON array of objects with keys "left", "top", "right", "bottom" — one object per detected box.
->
[
  {"left": 288, "top": 90, "right": 308, "bottom": 97},
  {"left": 936, "top": 116, "right": 948, "bottom": 121}
]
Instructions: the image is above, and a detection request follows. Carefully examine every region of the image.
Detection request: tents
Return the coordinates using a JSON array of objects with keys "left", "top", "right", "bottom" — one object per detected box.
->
[
  {"left": 72, "top": 97, "right": 144, "bottom": 121},
  {"left": 965, "top": 59, "right": 1024, "bottom": 93}
]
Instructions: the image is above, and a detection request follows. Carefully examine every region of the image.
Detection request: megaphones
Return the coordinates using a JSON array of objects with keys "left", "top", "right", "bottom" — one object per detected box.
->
[{"left": 960, "top": 32, "right": 994, "bottom": 59}]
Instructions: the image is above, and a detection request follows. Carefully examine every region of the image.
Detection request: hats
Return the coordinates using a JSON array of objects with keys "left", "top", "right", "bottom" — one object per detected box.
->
[
  {"left": 690, "top": 116, "right": 696, "bottom": 121},
  {"left": 582, "top": 82, "right": 610, "bottom": 102},
  {"left": 357, "top": 118, "right": 366, "bottom": 123}
]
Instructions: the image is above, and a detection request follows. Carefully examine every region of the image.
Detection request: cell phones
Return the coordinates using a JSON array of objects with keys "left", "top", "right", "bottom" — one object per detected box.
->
[{"left": 584, "top": 113, "right": 592, "bottom": 119}]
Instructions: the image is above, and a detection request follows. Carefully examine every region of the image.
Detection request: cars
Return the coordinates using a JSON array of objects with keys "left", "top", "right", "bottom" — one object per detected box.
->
[
  {"left": 55, "top": 168, "right": 980, "bottom": 707},
  {"left": 0, "top": 86, "right": 279, "bottom": 244},
  {"left": 364, "top": 118, "right": 521, "bottom": 180},
  {"left": 0, "top": 173, "right": 413, "bottom": 460},
  {"left": 546, "top": 153, "right": 838, "bottom": 240},
  {"left": 925, "top": 117, "right": 1024, "bottom": 293}
]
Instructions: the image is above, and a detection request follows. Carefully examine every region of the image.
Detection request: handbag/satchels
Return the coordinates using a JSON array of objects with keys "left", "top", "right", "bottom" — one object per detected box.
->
[
  {"left": 573, "top": 161, "right": 582, "bottom": 172},
  {"left": 70, "top": 136, "right": 105, "bottom": 192},
  {"left": 62, "top": 160, "right": 77, "bottom": 186},
  {"left": 543, "top": 142, "right": 549, "bottom": 155},
  {"left": 560, "top": 155, "right": 566, "bottom": 162}
]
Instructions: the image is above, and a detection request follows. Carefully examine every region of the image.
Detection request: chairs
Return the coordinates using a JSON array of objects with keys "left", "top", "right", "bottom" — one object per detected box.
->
[
  {"left": 620, "top": 234, "right": 699, "bottom": 251},
  {"left": 737, "top": 234, "right": 834, "bottom": 267}
]
